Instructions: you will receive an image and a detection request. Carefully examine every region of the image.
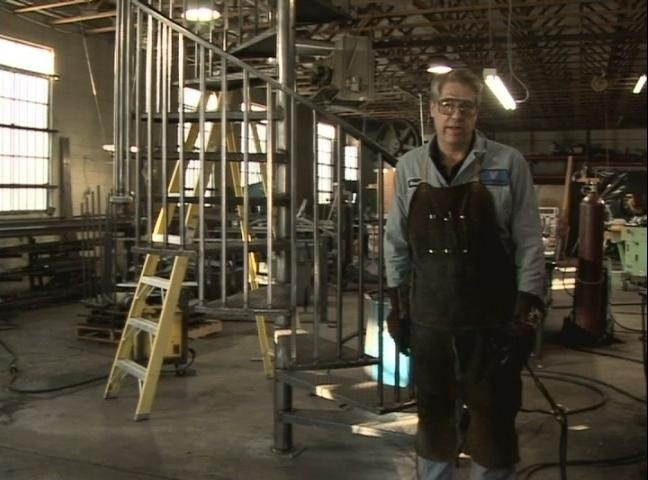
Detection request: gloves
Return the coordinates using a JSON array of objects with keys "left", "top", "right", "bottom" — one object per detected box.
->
[
  {"left": 385, "top": 304, "right": 411, "bottom": 357},
  {"left": 490, "top": 293, "right": 545, "bottom": 377}
]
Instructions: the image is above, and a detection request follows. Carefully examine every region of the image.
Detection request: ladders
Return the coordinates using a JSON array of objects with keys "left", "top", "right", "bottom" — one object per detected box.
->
[{"left": 103, "top": 87, "right": 301, "bottom": 422}]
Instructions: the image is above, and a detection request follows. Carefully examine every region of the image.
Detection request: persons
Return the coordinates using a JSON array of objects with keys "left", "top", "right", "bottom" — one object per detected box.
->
[{"left": 380, "top": 63, "right": 547, "bottom": 478}]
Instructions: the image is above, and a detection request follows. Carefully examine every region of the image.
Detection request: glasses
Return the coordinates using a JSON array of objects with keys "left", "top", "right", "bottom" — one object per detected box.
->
[{"left": 436, "top": 98, "right": 479, "bottom": 117}]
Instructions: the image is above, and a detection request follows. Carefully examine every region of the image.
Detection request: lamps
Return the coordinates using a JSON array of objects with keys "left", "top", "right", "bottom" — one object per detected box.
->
[
  {"left": 185, "top": 0, "right": 222, "bottom": 25},
  {"left": 425, "top": 46, "right": 452, "bottom": 74},
  {"left": 482, "top": 69, "right": 517, "bottom": 112},
  {"left": 633, "top": 74, "right": 648, "bottom": 95}
]
